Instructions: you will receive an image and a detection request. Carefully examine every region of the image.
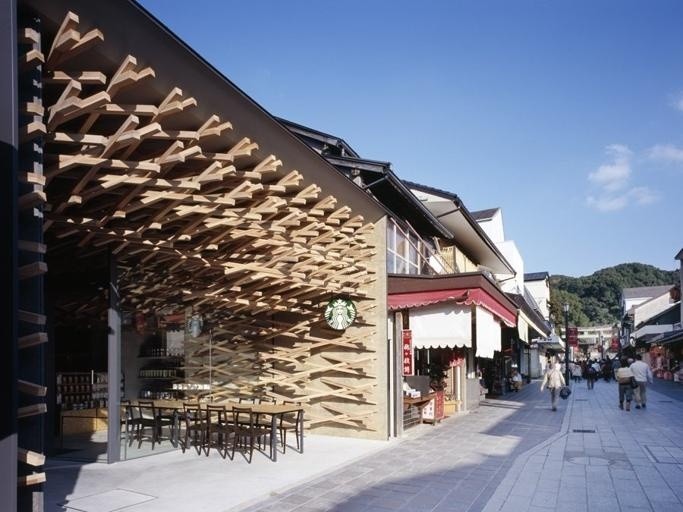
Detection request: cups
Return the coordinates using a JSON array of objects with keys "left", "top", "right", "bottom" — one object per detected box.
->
[
  {"left": 171, "top": 383, "right": 210, "bottom": 391},
  {"left": 139, "top": 391, "right": 172, "bottom": 399}
]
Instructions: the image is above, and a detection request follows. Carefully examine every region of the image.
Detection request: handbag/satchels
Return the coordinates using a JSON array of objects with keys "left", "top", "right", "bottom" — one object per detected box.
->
[
  {"left": 559, "top": 386, "right": 571, "bottom": 399},
  {"left": 630, "top": 376, "right": 638, "bottom": 388}
]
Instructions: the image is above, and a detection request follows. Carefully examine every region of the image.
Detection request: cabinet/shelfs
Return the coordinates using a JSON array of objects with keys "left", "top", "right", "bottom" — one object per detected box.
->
[
  {"left": 56, "top": 373, "right": 125, "bottom": 410},
  {"left": 135, "top": 357, "right": 184, "bottom": 400}
]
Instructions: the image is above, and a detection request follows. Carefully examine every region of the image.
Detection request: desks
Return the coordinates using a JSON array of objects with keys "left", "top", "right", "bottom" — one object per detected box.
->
[{"left": 404, "top": 395, "right": 434, "bottom": 424}]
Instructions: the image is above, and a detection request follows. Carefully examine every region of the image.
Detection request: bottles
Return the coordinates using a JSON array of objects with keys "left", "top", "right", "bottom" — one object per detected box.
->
[
  {"left": 140, "top": 347, "right": 184, "bottom": 357},
  {"left": 61, "top": 375, "right": 94, "bottom": 411},
  {"left": 139, "top": 366, "right": 176, "bottom": 378}
]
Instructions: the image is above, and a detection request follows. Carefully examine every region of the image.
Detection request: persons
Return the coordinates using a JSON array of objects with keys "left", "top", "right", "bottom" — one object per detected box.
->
[
  {"left": 540, "top": 352, "right": 680, "bottom": 411},
  {"left": 512, "top": 371, "right": 523, "bottom": 392}
]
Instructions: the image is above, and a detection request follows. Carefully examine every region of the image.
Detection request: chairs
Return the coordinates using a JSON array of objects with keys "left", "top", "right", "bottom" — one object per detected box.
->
[{"left": 121, "top": 396, "right": 302, "bottom": 464}]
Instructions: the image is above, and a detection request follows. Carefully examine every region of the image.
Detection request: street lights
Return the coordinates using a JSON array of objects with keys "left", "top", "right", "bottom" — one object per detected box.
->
[
  {"left": 562, "top": 299, "right": 570, "bottom": 385},
  {"left": 616, "top": 322, "right": 622, "bottom": 360},
  {"left": 601, "top": 335, "right": 604, "bottom": 359}
]
